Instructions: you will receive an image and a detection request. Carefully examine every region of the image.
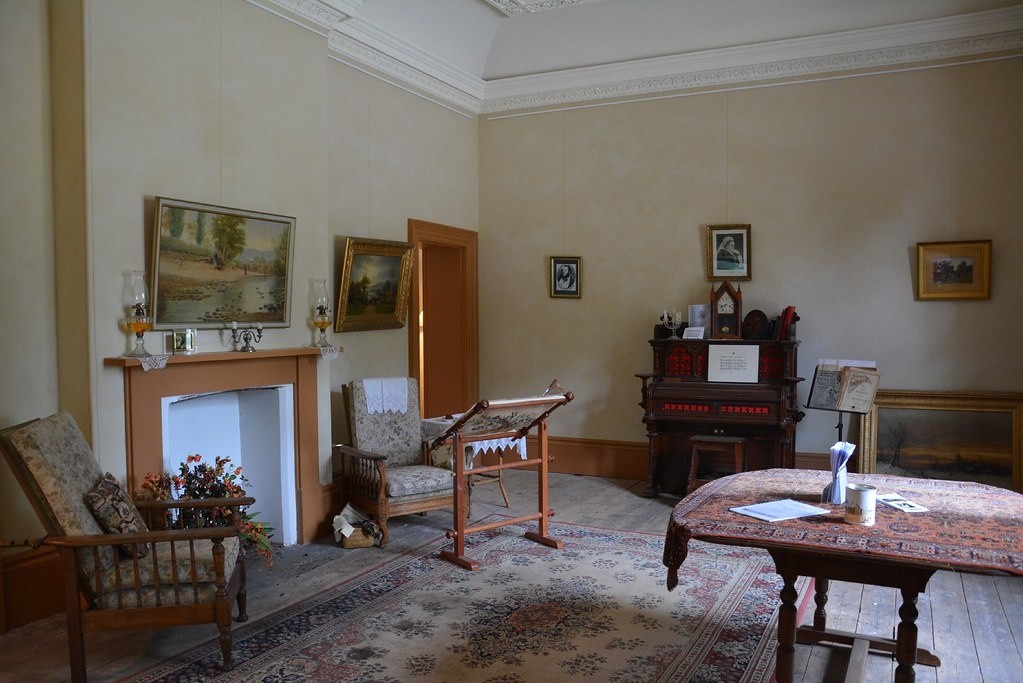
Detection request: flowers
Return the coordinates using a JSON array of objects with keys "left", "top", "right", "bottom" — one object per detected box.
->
[{"left": 141, "top": 452, "right": 275, "bottom": 570}]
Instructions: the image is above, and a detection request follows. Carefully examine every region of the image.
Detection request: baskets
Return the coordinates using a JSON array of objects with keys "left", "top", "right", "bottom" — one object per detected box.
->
[{"left": 342, "top": 525, "right": 375, "bottom": 549}]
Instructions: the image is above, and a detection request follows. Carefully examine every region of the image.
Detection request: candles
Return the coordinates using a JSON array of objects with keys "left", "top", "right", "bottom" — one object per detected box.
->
[
  {"left": 256, "top": 322, "right": 262, "bottom": 329},
  {"left": 232, "top": 321, "right": 237, "bottom": 330}
]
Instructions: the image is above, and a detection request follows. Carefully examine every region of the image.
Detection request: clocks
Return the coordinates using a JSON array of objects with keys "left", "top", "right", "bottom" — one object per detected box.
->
[{"left": 711, "top": 279, "right": 743, "bottom": 340}]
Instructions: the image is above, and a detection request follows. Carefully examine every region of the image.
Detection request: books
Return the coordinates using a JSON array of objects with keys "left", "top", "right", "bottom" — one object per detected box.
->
[
  {"left": 808, "top": 364, "right": 882, "bottom": 415},
  {"left": 728, "top": 498, "right": 832, "bottom": 523}
]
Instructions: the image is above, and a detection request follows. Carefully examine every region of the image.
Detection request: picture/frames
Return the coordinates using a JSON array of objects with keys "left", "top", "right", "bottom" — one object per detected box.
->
[
  {"left": 706, "top": 224, "right": 752, "bottom": 282},
  {"left": 173, "top": 331, "right": 195, "bottom": 351},
  {"left": 858, "top": 388, "right": 1023, "bottom": 495},
  {"left": 550, "top": 256, "right": 582, "bottom": 299},
  {"left": 333, "top": 237, "right": 412, "bottom": 333},
  {"left": 916, "top": 239, "right": 992, "bottom": 301},
  {"left": 151, "top": 195, "right": 297, "bottom": 330}
]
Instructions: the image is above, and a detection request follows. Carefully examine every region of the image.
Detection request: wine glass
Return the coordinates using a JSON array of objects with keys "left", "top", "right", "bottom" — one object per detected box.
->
[{"left": 663, "top": 309, "right": 682, "bottom": 340}]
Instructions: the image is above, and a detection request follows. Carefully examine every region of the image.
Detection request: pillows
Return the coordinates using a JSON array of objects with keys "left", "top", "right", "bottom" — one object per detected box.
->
[{"left": 87, "top": 471, "right": 151, "bottom": 559}]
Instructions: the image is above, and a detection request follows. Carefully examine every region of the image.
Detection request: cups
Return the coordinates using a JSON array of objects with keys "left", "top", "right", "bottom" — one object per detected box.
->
[{"left": 846, "top": 484, "right": 877, "bottom": 526}]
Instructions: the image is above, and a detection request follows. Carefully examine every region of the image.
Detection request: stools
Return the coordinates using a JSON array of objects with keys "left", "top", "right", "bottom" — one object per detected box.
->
[{"left": 684, "top": 434, "right": 748, "bottom": 498}]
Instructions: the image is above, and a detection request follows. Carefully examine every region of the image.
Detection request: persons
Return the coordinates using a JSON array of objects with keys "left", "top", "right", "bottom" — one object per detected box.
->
[
  {"left": 718, "top": 236, "right": 743, "bottom": 270},
  {"left": 557, "top": 265, "right": 575, "bottom": 289}
]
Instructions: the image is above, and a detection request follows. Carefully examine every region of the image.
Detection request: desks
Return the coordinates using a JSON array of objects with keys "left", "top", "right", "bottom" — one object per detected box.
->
[
  {"left": 662, "top": 468, "right": 1023, "bottom": 683},
  {"left": 421, "top": 412, "right": 528, "bottom": 508}
]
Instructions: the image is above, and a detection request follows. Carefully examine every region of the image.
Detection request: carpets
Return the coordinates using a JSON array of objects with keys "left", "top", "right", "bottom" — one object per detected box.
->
[{"left": 115, "top": 514, "right": 816, "bottom": 683}]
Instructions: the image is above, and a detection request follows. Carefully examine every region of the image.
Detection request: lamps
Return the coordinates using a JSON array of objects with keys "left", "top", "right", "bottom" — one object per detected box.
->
[
  {"left": 312, "top": 279, "right": 332, "bottom": 347},
  {"left": 127, "top": 270, "right": 152, "bottom": 356}
]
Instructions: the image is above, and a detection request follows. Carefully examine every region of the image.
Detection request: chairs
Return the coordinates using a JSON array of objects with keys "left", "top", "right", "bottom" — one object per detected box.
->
[
  {"left": 333, "top": 377, "right": 473, "bottom": 549},
  {"left": 0, "top": 411, "right": 256, "bottom": 683}
]
